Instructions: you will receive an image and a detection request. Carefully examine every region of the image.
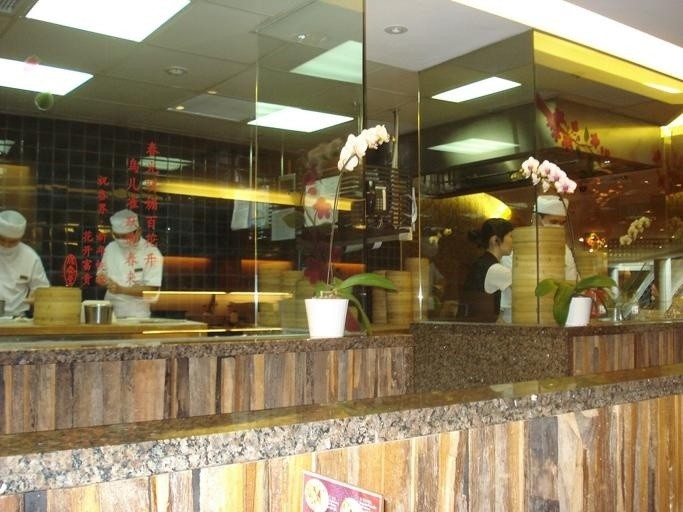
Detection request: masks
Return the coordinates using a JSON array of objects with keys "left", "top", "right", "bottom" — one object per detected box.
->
[
  {"left": 541, "top": 220, "right": 565, "bottom": 228},
  {"left": 0, "top": 245, "right": 19, "bottom": 256},
  {"left": 114, "top": 234, "right": 131, "bottom": 248}
]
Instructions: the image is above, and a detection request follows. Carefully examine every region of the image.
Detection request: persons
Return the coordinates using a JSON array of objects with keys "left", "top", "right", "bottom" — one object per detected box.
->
[
  {"left": 0, "top": 208, "right": 50, "bottom": 320},
  {"left": 456, "top": 215, "right": 514, "bottom": 322},
  {"left": 97, "top": 209, "right": 163, "bottom": 322},
  {"left": 497, "top": 193, "right": 582, "bottom": 326}
]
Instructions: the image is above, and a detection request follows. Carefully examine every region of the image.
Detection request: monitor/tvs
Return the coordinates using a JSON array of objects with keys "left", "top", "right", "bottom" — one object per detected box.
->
[{"left": 303, "top": 173, "right": 340, "bottom": 229}]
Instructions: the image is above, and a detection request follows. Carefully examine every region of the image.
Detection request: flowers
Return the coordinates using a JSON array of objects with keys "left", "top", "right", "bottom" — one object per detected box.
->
[
  {"left": 521, "top": 156, "right": 617, "bottom": 326},
  {"left": 310, "top": 122, "right": 398, "bottom": 333},
  {"left": 619, "top": 208, "right": 652, "bottom": 248}
]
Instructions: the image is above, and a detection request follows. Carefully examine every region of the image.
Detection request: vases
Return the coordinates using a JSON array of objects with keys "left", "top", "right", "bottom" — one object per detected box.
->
[
  {"left": 305, "top": 300, "right": 349, "bottom": 340},
  {"left": 566, "top": 297, "right": 593, "bottom": 328}
]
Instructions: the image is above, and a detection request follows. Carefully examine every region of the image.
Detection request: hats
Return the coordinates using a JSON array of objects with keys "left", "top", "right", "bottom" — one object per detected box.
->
[
  {"left": 111, "top": 210, "right": 139, "bottom": 234},
  {"left": 534, "top": 193, "right": 568, "bottom": 217},
  {"left": 0, "top": 210, "right": 27, "bottom": 240}
]
